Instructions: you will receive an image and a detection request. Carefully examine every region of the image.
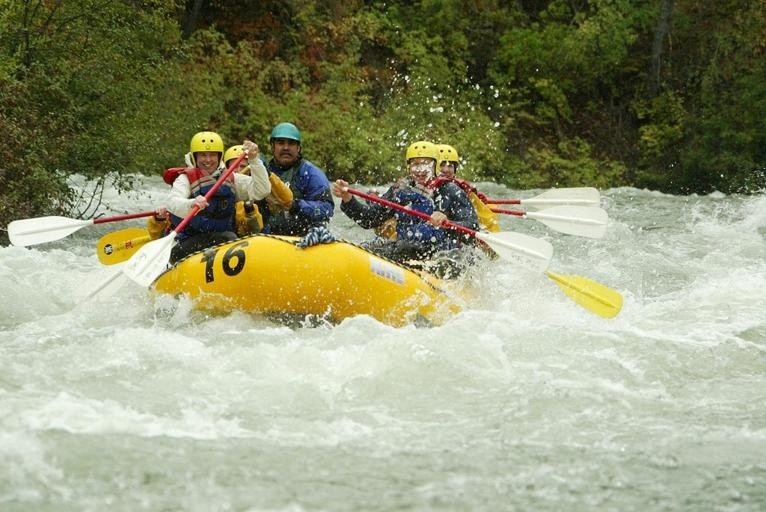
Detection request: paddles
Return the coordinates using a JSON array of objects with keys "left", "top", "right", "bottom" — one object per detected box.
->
[
  {"left": 121, "top": 148, "right": 257, "bottom": 288},
  {"left": 546, "top": 269, "right": 623, "bottom": 319},
  {"left": 337, "top": 179, "right": 554, "bottom": 276},
  {"left": 485, "top": 187, "right": 609, "bottom": 239},
  {"left": 97, "top": 228, "right": 152, "bottom": 266}
]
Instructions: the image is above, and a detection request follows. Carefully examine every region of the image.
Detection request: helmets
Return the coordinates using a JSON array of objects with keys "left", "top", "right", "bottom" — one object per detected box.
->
[
  {"left": 270, "top": 122, "right": 301, "bottom": 144},
  {"left": 190, "top": 131, "right": 225, "bottom": 168},
  {"left": 436, "top": 143, "right": 460, "bottom": 175},
  {"left": 225, "top": 144, "right": 245, "bottom": 170},
  {"left": 406, "top": 140, "right": 441, "bottom": 176}
]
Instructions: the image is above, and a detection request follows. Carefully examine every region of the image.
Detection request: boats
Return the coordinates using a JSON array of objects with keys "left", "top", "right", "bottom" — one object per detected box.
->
[{"left": 134, "top": 232, "right": 473, "bottom": 329}]
